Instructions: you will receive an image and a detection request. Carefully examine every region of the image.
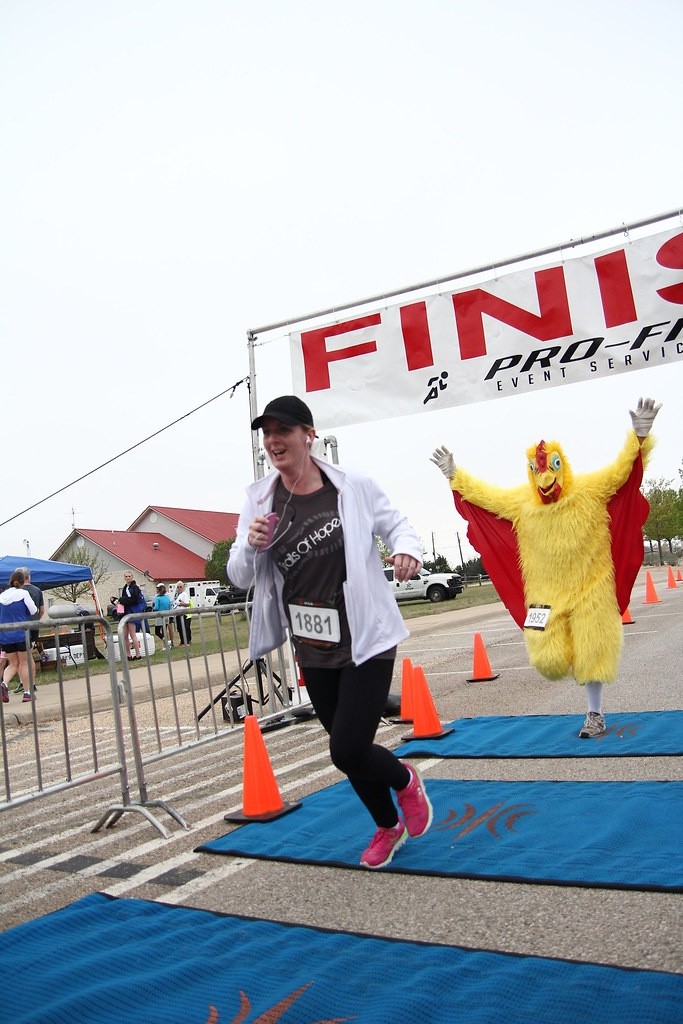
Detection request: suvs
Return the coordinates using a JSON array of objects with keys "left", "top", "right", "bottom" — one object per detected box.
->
[
  {"left": 218, "top": 588, "right": 253, "bottom": 614},
  {"left": 383, "top": 565, "right": 465, "bottom": 603}
]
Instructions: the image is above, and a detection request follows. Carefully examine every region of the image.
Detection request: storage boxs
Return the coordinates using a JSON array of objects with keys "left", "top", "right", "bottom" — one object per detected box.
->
[
  {"left": 31, "top": 633, "right": 85, "bottom": 672},
  {"left": 104, "top": 632, "right": 155, "bottom": 661}
]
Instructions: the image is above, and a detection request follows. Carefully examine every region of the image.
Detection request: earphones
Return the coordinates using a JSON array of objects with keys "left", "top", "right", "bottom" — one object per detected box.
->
[{"left": 306, "top": 435, "right": 310, "bottom": 443}]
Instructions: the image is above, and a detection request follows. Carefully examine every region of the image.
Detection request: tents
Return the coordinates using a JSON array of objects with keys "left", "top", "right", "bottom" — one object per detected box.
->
[{"left": 0, "top": 555, "right": 104, "bottom": 617}]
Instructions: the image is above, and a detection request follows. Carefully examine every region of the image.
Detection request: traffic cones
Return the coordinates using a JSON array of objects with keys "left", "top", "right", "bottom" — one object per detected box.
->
[
  {"left": 465, "top": 632, "right": 500, "bottom": 682},
  {"left": 666, "top": 566, "right": 679, "bottom": 589},
  {"left": 621, "top": 608, "right": 636, "bottom": 624},
  {"left": 400, "top": 667, "right": 456, "bottom": 741},
  {"left": 641, "top": 572, "right": 662, "bottom": 604},
  {"left": 675, "top": 568, "right": 683, "bottom": 581},
  {"left": 389, "top": 657, "right": 415, "bottom": 725},
  {"left": 223, "top": 715, "right": 303, "bottom": 824}
]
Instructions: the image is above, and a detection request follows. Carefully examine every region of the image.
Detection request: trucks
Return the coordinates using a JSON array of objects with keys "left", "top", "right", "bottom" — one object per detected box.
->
[{"left": 169, "top": 580, "right": 229, "bottom": 609}]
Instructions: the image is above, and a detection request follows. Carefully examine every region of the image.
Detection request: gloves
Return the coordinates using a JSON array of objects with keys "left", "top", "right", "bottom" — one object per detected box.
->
[
  {"left": 430, "top": 445, "right": 457, "bottom": 478},
  {"left": 628, "top": 396, "right": 663, "bottom": 437}
]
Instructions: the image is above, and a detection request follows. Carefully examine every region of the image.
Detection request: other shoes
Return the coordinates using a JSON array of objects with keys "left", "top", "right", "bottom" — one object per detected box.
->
[{"left": 161, "top": 640, "right": 191, "bottom": 651}]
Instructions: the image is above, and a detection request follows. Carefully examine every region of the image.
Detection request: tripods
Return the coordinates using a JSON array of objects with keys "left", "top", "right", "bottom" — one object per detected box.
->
[{"left": 194, "top": 656, "right": 292, "bottom": 721}]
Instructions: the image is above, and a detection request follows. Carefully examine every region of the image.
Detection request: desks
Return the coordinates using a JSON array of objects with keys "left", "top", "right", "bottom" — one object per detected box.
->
[{"left": 37, "top": 633, "right": 79, "bottom": 669}]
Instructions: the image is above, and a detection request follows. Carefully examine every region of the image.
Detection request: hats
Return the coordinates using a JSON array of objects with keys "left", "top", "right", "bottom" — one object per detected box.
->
[
  {"left": 251, "top": 396, "right": 314, "bottom": 430},
  {"left": 156, "top": 583, "right": 165, "bottom": 588}
]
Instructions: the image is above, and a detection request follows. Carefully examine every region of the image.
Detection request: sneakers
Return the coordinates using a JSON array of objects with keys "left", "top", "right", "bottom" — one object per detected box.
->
[
  {"left": 392, "top": 761, "right": 433, "bottom": 840},
  {"left": 22, "top": 692, "right": 37, "bottom": 701},
  {"left": 360, "top": 816, "right": 410, "bottom": 870},
  {"left": 128, "top": 655, "right": 132, "bottom": 662},
  {"left": 14, "top": 683, "right": 24, "bottom": 693},
  {"left": 34, "top": 685, "right": 38, "bottom": 691},
  {"left": 578, "top": 711, "right": 606, "bottom": 738},
  {"left": 129, "top": 655, "right": 142, "bottom": 661},
  {"left": 1, "top": 683, "right": 9, "bottom": 702}
]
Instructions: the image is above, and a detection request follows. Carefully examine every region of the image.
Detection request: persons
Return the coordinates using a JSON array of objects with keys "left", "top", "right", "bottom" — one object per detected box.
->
[
  {"left": 225, "top": 396, "right": 434, "bottom": 869},
  {"left": 0, "top": 567, "right": 44, "bottom": 703},
  {"left": 172, "top": 580, "right": 194, "bottom": 646},
  {"left": 118, "top": 572, "right": 143, "bottom": 661},
  {"left": 150, "top": 583, "right": 176, "bottom": 651},
  {"left": 74, "top": 605, "right": 105, "bottom": 660},
  {"left": 430, "top": 396, "right": 663, "bottom": 738},
  {"left": 135, "top": 590, "right": 151, "bottom": 633}
]
